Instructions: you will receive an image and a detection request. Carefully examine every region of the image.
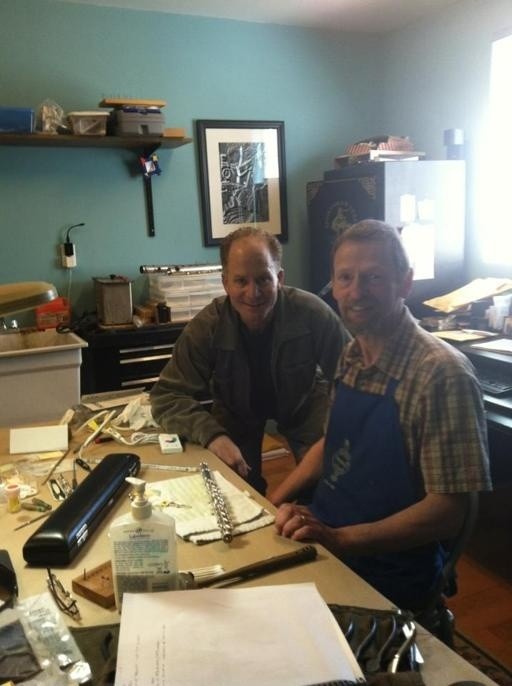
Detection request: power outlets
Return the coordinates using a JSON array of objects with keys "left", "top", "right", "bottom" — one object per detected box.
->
[{"left": 59, "top": 240, "right": 78, "bottom": 271}]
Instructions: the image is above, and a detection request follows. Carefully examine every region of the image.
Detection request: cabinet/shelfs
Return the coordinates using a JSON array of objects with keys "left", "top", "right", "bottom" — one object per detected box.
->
[
  {"left": 302, "top": 160, "right": 465, "bottom": 319},
  {"left": 94, "top": 328, "right": 182, "bottom": 390}
]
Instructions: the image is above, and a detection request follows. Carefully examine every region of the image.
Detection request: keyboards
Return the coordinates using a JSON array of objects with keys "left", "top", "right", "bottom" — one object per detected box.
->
[{"left": 479, "top": 377, "right": 511, "bottom": 397}]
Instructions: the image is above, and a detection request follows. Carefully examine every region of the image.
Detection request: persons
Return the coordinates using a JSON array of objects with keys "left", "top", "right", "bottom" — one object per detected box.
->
[
  {"left": 266, "top": 215, "right": 497, "bottom": 650},
  {"left": 147, "top": 223, "right": 358, "bottom": 505}
]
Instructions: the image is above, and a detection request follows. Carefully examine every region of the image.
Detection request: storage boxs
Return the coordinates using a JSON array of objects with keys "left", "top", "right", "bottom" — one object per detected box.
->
[{"left": 146, "top": 272, "right": 227, "bottom": 324}]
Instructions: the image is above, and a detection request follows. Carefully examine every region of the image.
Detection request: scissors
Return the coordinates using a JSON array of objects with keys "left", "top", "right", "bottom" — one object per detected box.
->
[{"left": 49, "top": 480, "right": 65, "bottom": 499}]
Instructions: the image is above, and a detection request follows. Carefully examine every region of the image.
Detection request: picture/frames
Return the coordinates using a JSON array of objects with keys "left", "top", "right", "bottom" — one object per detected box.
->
[{"left": 194, "top": 117, "right": 291, "bottom": 247}]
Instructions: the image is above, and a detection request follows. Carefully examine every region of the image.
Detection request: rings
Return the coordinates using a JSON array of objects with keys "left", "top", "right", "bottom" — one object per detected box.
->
[{"left": 300, "top": 515, "right": 307, "bottom": 524}]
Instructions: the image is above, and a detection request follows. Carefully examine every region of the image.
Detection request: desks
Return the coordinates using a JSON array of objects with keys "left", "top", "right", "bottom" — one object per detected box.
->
[{"left": 0, "top": 415, "right": 512, "bottom": 686}]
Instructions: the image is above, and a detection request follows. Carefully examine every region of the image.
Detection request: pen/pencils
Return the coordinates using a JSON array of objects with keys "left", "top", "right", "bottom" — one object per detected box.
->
[
  {"left": 12, "top": 511, "right": 53, "bottom": 532},
  {"left": 95, "top": 438, "right": 113, "bottom": 443}
]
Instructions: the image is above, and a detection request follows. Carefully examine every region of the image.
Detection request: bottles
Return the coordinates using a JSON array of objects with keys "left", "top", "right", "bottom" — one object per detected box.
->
[{"left": 440, "top": 127, "right": 465, "bottom": 160}]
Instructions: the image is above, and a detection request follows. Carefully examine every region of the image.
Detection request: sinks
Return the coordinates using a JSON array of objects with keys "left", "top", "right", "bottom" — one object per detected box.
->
[{"left": 0, "top": 329, "right": 87, "bottom": 425}]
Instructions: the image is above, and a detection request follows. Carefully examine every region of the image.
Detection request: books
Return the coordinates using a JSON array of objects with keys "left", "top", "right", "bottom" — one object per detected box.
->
[
  {"left": 335, "top": 148, "right": 426, "bottom": 159},
  {"left": 470, "top": 335, "right": 512, "bottom": 357},
  {"left": 97, "top": 94, "right": 167, "bottom": 107},
  {"left": 9, "top": 422, "right": 69, "bottom": 455},
  {"left": 356, "top": 155, "right": 419, "bottom": 162}
]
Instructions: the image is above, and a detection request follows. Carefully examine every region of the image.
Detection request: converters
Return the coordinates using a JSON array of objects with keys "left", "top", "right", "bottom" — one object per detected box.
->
[{"left": 65, "top": 242, "right": 73, "bottom": 257}]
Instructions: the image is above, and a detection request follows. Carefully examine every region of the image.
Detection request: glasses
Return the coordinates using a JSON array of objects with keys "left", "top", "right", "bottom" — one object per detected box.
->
[{"left": 47, "top": 568, "right": 79, "bottom": 617}]
